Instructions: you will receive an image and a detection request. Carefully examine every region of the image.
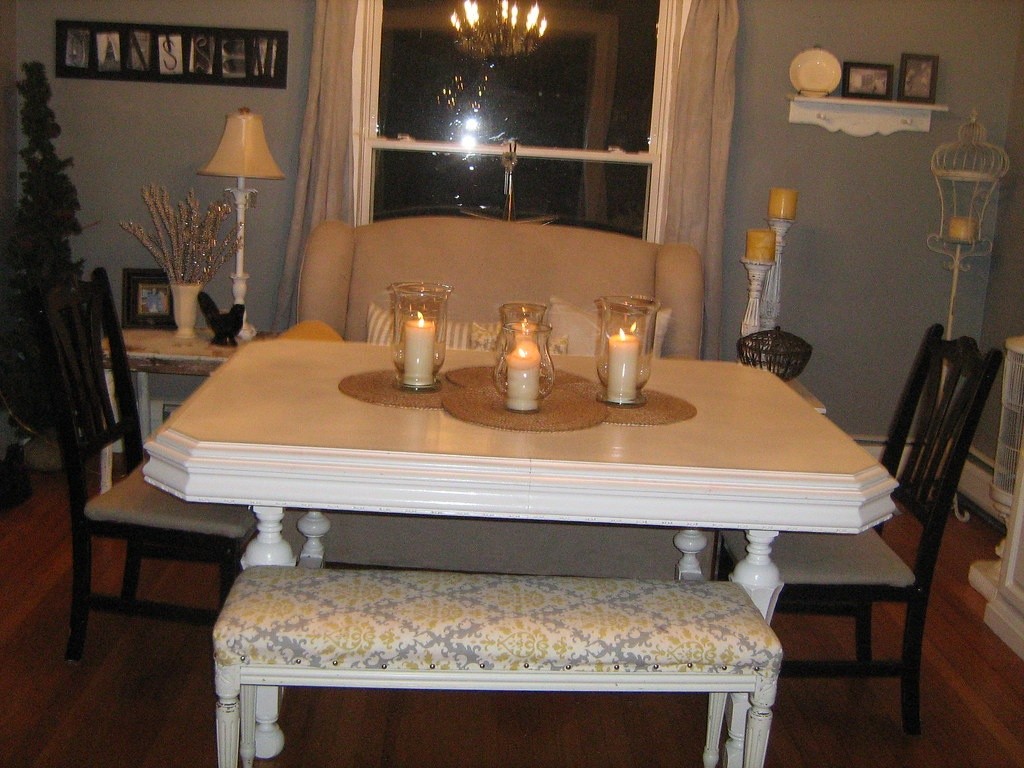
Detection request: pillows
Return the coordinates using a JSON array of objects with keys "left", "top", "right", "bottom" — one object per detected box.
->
[
  {"left": 548, "top": 293, "right": 673, "bottom": 358},
  {"left": 366, "top": 299, "right": 473, "bottom": 349},
  {"left": 272, "top": 319, "right": 346, "bottom": 342},
  {"left": 471, "top": 320, "right": 572, "bottom": 357}
]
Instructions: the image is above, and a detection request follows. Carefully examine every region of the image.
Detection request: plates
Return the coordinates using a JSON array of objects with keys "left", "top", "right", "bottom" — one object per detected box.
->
[{"left": 790, "top": 50, "right": 842, "bottom": 97}]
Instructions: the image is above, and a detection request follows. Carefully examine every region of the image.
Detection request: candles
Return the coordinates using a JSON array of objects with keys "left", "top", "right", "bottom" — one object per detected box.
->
[
  {"left": 607, "top": 327, "right": 638, "bottom": 403},
  {"left": 767, "top": 187, "right": 798, "bottom": 219},
  {"left": 950, "top": 215, "right": 979, "bottom": 240},
  {"left": 506, "top": 345, "right": 539, "bottom": 412},
  {"left": 744, "top": 228, "right": 776, "bottom": 263},
  {"left": 403, "top": 312, "right": 434, "bottom": 386}
]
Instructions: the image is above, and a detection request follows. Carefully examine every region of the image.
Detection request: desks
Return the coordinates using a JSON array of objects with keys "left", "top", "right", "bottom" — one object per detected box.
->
[{"left": 141, "top": 337, "right": 900, "bottom": 768}]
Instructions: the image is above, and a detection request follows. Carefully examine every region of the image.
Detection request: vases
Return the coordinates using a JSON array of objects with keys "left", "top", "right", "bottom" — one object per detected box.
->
[{"left": 170, "top": 282, "right": 201, "bottom": 342}]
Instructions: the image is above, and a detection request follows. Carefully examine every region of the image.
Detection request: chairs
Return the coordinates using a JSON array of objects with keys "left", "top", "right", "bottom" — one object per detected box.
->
[
  {"left": 697, "top": 323, "right": 1001, "bottom": 741},
  {"left": 22, "top": 269, "right": 258, "bottom": 663}
]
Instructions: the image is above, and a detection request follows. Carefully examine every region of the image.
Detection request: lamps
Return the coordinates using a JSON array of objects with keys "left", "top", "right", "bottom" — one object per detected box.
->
[
  {"left": 194, "top": 105, "right": 287, "bottom": 342},
  {"left": 449, "top": 0, "right": 549, "bottom": 65}
]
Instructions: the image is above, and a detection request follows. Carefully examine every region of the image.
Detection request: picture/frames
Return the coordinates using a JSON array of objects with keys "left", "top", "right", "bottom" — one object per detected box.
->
[
  {"left": 840, "top": 61, "right": 893, "bottom": 102},
  {"left": 54, "top": 18, "right": 289, "bottom": 90},
  {"left": 895, "top": 52, "right": 939, "bottom": 105},
  {"left": 119, "top": 267, "right": 178, "bottom": 330}
]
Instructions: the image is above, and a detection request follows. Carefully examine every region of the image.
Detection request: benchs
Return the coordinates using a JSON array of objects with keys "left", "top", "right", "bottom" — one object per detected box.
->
[
  {"left": 210, "top": 565, "right": 783, "bottom": 768},
  {"left": 299, "top": 213, "right": 705, "bottom": 576}
]
